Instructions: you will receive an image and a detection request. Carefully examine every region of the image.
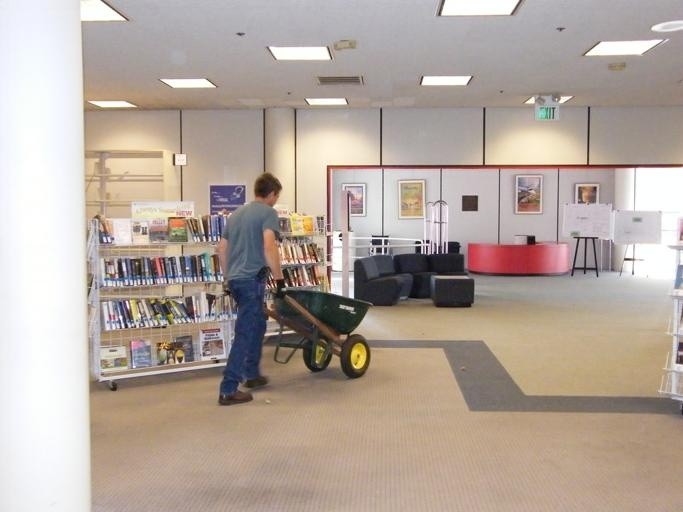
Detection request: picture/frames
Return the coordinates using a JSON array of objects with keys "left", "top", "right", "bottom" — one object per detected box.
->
[
  {"left": 341, "top": 182, "right": 368, "bottom": 218},
  {"left": 397, "top": 178, "right": 426, "bottom": 221},
  {"left": 574, "top": 182, "right": 600, "bottom": 207},
  {"left": 513, "top": 173, "right": 544, "bottom": 216}
]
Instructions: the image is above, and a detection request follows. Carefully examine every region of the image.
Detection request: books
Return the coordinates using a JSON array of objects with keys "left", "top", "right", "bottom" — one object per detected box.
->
[
  {"left": 156, "top": 343, "right": 185, "bottom": 364},
  {"left": 277, "top": 208, "right": 326, "bottom": 236},
  {"left": 199, "top": 329, "right": 227, "bottom": 360},
  {"left": 99, "top": 291, "right": 220, "bottom": 332},
  {"left": 97, "top": 248, "right": 218, "bottom": 289},
  {"left": 99, "top": 345, "right": 128, "bottom": 374},
  {"left": 148, "top": 216, "right": 189, "bottom": 244},
  {"left": 187, "top": 212, "right": 233, "bottom": 244},
  {"left": 264, "top": 265, "right": 324, "bottom": 289},
  {"left": 276, "top": 238, "right": 323, "bottom": 264},
  {"left": 173, "top": 335, "right": 194, "bottom": 362},
  {"left": 220, "top": 289, "right": 237, "bottom": 322},
  {"left": 93, "top": 214, "right": 114, "bottom": 245},
  {"left": 130, "top": 339, "right": 151, "bottom": 369}
]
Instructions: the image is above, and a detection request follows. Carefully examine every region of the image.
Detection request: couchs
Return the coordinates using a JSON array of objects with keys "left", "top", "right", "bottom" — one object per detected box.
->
[
  {"left": 353, "top": 254, "right": 414, "bottom": 307},
  {"left": 393, "top": 253, "right": 438, "bottom": 299},
  {"left": 467, "top": 241, "right": 572, "bottom": 275},
  {"left": 429, "top": 254, "right": 468, "bottom": 275}
]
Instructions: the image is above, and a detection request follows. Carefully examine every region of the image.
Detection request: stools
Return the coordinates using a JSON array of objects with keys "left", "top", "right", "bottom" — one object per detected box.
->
[{"left": 430, "top": 276, "right": 475, "bottom": 308}]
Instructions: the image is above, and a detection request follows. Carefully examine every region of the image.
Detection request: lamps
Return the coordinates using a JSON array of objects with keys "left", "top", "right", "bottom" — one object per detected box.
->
[{"left": 534, "top": 94, "right": 561, "bottom": 122}]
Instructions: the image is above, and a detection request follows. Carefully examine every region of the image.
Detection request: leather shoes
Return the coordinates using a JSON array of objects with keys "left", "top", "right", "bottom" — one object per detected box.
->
[
  {"left": 243, "top": 374, "right": 267, "bottom": 388},
  {"left": 219, "top": 390, "right": 253, "bottom": 406}
]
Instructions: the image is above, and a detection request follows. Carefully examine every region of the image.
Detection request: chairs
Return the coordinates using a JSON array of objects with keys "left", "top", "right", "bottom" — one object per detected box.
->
[
  {"left": 371, "top": 235, "right": 389, "bottom": 254},
  {"left": 445, "top": 241, "right": 461, "bottom": 253},
  {"left": 415, "top": 240, "right": 431, "bottom": 254}
]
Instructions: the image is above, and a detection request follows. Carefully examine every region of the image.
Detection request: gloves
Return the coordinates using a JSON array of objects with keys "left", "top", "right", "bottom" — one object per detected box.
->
[
  {"left": 276, "top": 279, "right": 286, "bottom": 300},
  {"left": 223, "top": 279, "right": 230, "bottom": 295}
]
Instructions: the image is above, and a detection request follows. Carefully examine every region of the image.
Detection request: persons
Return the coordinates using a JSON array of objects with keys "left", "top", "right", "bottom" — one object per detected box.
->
[{"left": 219, "top": 171, "right": 288, "bottom": 406}]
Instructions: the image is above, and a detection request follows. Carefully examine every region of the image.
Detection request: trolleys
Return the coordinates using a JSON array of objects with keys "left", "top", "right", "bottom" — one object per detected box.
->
[{"left": 266, "top": 290, "right": 374, "bottom": 378}]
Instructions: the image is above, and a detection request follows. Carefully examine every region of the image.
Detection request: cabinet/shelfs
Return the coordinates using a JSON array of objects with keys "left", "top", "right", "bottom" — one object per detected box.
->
[
  {"left": 657, "top": 216, "right": 683, "bottom": 417},
  {"left": 85, "top": 212, "right": 237, "bottom": 392},
  {"left": 264, "top": 206, "right": 333, "bottom": 340},
  {"left": 85, "top": 147, "right": 178, "bottom": 218}
]
